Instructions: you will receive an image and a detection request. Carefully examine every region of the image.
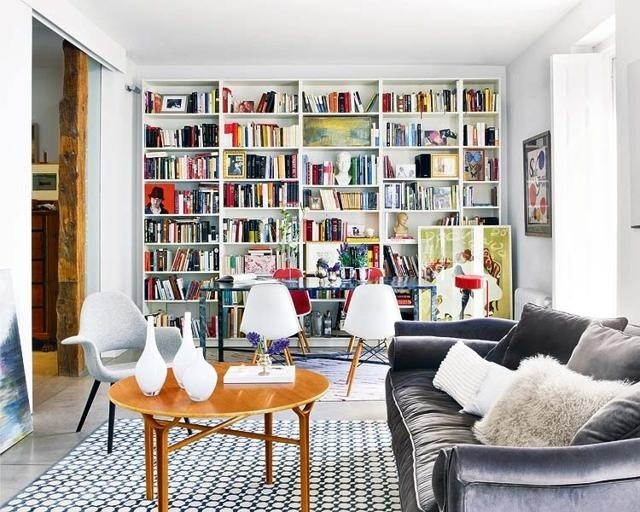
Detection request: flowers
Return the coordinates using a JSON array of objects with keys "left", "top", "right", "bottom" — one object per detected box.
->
[{"left": 247, "top": 332, "right": 290, "bottom": 354}]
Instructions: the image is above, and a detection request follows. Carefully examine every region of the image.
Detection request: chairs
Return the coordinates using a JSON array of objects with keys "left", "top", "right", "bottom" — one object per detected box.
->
[
  {"left": 272, "top": 268, "right": 312, "bottom": 357},
  {"left": 240, "top": 284, "right": 302, "bottom": 366},
  {"left": 342, "top": 288, "right": 355, "bottom": 353},
  {"left": 61, "top": 291, "right": 193, "bottom": 454},
  {"left": 341, "top": 284, "right": 403, "bottom": 397}
]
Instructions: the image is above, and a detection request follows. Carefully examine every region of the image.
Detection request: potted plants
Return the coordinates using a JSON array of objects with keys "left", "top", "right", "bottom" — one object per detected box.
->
[
  {"left": 354, "top": 254, "right": 371, "bottom": 283},
  {"left": 338, "top": 251, "right": 354, "bottom": 280}
]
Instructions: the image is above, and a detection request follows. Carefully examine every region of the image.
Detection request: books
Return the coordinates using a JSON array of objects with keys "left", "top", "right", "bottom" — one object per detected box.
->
[
  {"left": 222, "top": 87, "right": 299, "bottom": 338},
  {"left": 143, "top": 87, "right": 218, "bottom": 338},
  {"left": 393, "top": 287, "right": 412, "bottom": 306},
  {"left": 302, "top": 90, "right": 379, "bottom": 299},
  {"left": 383, "top": 87, "right": 459, "bottom": 278},
  {"left": 462, "top": 87, "right": 500, "bottom": 226}
]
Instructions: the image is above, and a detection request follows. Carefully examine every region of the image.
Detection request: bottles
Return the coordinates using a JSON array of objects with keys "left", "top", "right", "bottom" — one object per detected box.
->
[
  {"left": 339, "top": 311, "right": 347, "bottom": 331},
  {"left": 304, "top": 314, "right": 311, "bottom": 338},
  {"left": 323, "top": 310, "right": 332, "bottom": 338},
  {"left": 311, "top": 311, "right": 323, "bottom": 337}
]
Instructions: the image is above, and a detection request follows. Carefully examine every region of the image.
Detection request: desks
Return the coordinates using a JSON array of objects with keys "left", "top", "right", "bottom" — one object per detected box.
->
[
  {"left": 107, "top": 362, "right": 331, "bottom": 512},
  {"left": 200, "top": 275, "right": 437, "bottom": 362}
]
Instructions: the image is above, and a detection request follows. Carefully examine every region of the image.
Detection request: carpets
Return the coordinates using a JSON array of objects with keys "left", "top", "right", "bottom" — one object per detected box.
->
[{"left": 0, "top": 418, "right": 403, "bottom": 512}]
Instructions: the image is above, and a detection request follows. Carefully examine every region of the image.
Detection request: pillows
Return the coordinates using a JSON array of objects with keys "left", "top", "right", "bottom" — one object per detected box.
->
[{"left": 472, "top": 302, "right": 640, "bottom": 446}]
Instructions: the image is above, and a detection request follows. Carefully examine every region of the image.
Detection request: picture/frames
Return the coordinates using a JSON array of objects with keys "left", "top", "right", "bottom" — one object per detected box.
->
[
  {"left": 418, "top": 225, "right": 513, "bottom": 322},
  {"left": 522, "top": 130, "right": 552, "bottom": 237}
]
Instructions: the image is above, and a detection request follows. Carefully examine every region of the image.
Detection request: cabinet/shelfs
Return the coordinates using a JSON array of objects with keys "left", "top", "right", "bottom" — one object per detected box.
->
[
  {"left": 141, "top": 77, "right": 501, "bottom": 346},
  {"left": 32, "top": 208, "right": 57, "bottom": 346}
]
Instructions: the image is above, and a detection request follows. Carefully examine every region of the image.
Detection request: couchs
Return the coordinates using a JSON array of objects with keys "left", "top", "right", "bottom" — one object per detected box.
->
[{"left": 385, "top": 318, "right": 640, "bottom": 512}]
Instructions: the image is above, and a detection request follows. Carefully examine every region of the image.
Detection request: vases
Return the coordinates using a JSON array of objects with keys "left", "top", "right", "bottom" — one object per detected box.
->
[
  {"left": 134, "top": 316, "right": 168, "bottom": 398},
  {"left": 172, "top": 311, "right": 195, "bottom": 391},
  {"left": 257, "top": 354, "right": 272, "bottom": 376},
  {"left": 184, "top": 346, "right": 217, "bottom": 403}
]
